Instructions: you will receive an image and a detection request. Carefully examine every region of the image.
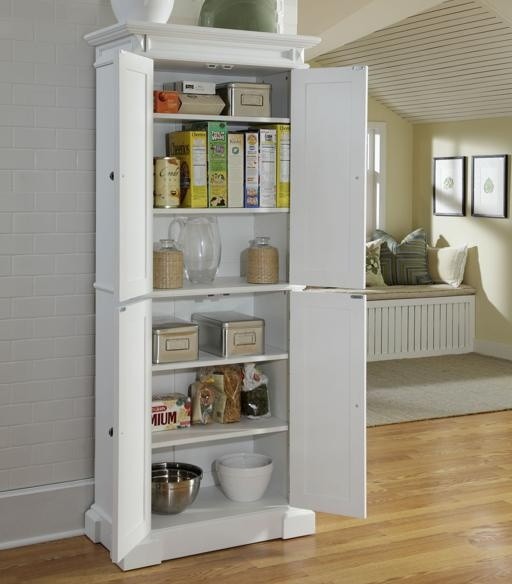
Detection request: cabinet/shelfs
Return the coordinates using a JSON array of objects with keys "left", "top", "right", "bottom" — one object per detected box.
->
[{"left": 83, "top": 20, "right": 366, "bottom": 570}]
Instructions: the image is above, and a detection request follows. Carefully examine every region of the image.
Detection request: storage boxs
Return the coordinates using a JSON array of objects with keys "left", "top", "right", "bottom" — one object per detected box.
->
[
  {"left": 215, "top": 82, "right": 271, "bottom": 117},
  {"left": 152, "top": 316, "right": 200, "bottom": 364},
  {"left": 191, "top": 312, "right": 265, "bottom": 358}
]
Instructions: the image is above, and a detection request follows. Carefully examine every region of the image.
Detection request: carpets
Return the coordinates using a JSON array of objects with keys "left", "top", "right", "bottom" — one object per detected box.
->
[{"left": 367, "top": 352, "right": 512, "bottom": 428}]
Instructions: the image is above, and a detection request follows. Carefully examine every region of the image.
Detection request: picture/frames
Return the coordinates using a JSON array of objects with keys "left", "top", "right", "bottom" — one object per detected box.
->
[
  {"left": 432, "top": 156, "right": 466, "bottom": 216},
  {"left": 472, "top": 155, "right": 507, "bottom": 218}
]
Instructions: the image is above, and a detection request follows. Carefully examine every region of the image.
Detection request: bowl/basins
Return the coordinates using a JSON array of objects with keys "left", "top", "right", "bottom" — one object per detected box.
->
[
  {"left": 153, "top": 463, "right": 204, "bottom": 516},
  {"left": 216, "top": 453, "right": 276, "bottom": 502}
]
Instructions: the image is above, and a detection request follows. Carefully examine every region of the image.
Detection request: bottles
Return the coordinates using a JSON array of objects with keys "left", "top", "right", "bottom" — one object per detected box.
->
[
  {"left": 153, "top": 240, "right": 185, "bottom": 289},
  {"left": 246, "top": 237, "right": 279, "bottom": 284}
]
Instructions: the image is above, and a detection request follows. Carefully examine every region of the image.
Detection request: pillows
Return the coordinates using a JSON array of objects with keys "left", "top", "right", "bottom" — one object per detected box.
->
[
  {"left": 366, "top": 239, "right": 385, "bottom": 286},
  {"left": 426, "top": 245, "right": 468, "bottom": 287},
  {"left": 373, "top": 227, "right": 432, "bottom": 284}
]
